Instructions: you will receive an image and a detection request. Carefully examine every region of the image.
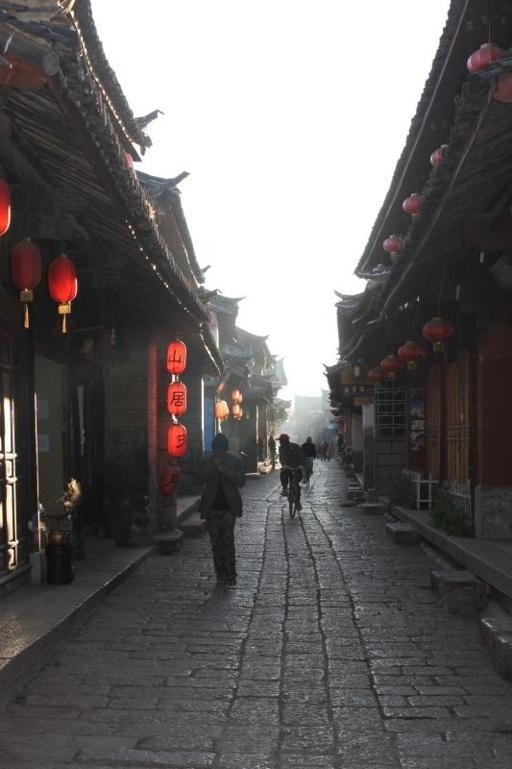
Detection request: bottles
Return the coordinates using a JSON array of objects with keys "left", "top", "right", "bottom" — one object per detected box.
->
[{"left": 275, "top": 434, "right": 289, "bottom": 441}]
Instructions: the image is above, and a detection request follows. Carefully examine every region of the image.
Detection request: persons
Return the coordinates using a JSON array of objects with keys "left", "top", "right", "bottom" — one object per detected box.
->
[
  {"left": 277, "top": 433, "right": 306, "bottom": 512},
  {"left": 196, "top": 434, "right": 245, "bottom": 589},
  {"left": 268, "top": 436, "right": 275, "bottom": 458},
  {"left": 337, "top": 433, "right": 343, "bottom": 449},
  {"left": 302, "top": 437, "right": 316, "bottom": 482},
  {"left": 318, "top": 437, "right": 334, "bottom": 462}
]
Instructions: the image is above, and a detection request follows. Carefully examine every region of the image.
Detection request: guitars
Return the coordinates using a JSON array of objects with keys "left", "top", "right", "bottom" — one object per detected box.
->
[
  {"left": 165, "top": 337, "right": 189, "bottom": 457},
  {"left": 11, "top": 238, "right": 42, "bottom": 329},
  {"left": 215, "top": 383, "right": 243, "bottom": 422},
  {"left": 47, "top": 253, "right": 78, "bottom": 333},
  {"left": 366, "top": 340, "right": 427, "bottom": 381},
  {"left": 430, "top": 148, "right": 443, "bottom": 166},
  {"left": 402, "top": 193, "right": 422, "bottom": 224},
  {"left": 422, "top": 316, "right": 456, "bottom": 353},
  {"left": 383, "top": 234, "right": 400, "bottom": 264},
  {"left": 465, "top": 43, "right": 503, "bottom": 73},
  {"left": 0, "top": 179, "right": 12, "bottom": 235}
]
